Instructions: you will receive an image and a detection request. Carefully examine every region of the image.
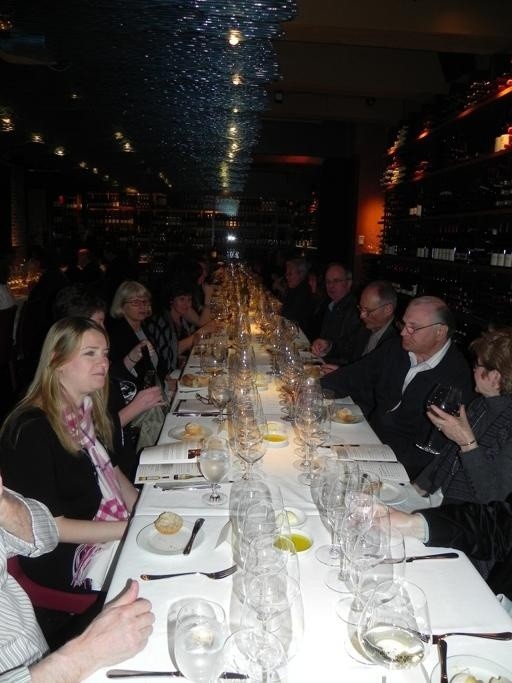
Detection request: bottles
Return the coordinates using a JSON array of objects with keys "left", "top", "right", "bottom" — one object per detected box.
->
[
  {"left": 50, "top": 189, "right": 321, "bottom": 267},
  {"left": 376, "top": 70, "right": 512, "bottom": 263}
]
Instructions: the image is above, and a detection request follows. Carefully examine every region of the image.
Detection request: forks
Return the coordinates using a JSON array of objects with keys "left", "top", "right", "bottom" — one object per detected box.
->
[
  {"left": 140, "top": 564, "right": 238, "bottom": 581},
  {"left": 406, "top": 627, "right": 512, "bottom": 645}
]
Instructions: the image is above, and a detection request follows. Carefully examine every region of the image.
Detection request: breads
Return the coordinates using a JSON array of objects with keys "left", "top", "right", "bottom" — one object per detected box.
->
[
  {"left": 336, "top": 407, "right": 353, "bottom": 421},
  {"left": 304, "top": 366, "right": 321, "bottom": 378},
  {"left": 182, "top": 374, "right": 210, "bottom": 390},
  {"left": 153, "top": 511, "right": 184, "bottom": 535},
  {"left": 186, "top": 421, "right": 202, "bottom": 438}
]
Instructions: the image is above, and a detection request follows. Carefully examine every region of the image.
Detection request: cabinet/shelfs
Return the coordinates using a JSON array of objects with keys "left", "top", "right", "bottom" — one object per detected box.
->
[{"left": 382, "top": 84, "right": 512, "bottom": 330}]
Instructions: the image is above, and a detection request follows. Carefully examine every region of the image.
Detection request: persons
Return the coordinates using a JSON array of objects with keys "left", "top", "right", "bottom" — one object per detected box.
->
[{"left": 0, "top": 241, "right": 512, "bottom": 683}]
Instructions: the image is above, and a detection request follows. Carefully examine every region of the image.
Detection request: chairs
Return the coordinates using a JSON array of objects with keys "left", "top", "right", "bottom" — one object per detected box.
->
[{"left": 7, "top": 556, "right": 107, "bottom": 614}]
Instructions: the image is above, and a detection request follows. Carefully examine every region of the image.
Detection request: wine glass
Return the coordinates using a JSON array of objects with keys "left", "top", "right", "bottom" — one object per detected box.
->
[
  {"left": 171, "top": 250, "right": 431, "bottom": 683},
  {"left": 413, "top": 383, "right": 463, "bottom": 457}
]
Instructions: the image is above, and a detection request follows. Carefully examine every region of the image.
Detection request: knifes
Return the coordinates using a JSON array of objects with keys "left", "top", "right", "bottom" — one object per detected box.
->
[
  {"left": 106, "top": 669, "right": 250, "bottom": 680},
  {"left": 373, "top": 553, "right": 459, "bottom": 564},
  {"left": 153, "top": 481, "right": 234, "bottom": 491}
]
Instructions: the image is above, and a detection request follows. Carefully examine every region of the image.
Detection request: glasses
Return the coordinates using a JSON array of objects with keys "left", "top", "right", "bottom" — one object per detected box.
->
[
  {"left": 124, "top": 300, "right": 150, "bottom": 307},
  {"left": 354, "top": 302, "right": 393, "bottom": 315},
  {"left": 325, "top": 279, "right": 347, "bottom": 286},
  {"left": 395, "top": 323, "right": 445, "bottom": 334}
]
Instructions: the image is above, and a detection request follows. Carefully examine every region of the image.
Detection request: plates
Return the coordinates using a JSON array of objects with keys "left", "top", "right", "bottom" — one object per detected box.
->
[
  {"left": 430, "top": 655, "right": 512, "bottom": 683},
  {"left": 167, "top": 424, "right": 214, "bottom": 442},
  {"left": 136, "top": 520, "right": 205, "bottom": 556}
]
